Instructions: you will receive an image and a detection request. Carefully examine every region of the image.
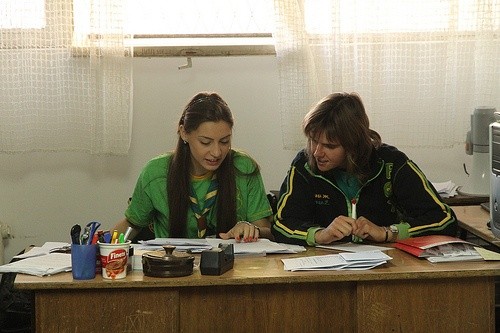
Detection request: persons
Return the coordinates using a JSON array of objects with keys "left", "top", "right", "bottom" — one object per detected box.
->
[
  {"left": 271, "top": 89, "right": 459, "bottom": 249},
  {"left": 106, "top": 92, "right": 274, "bottom": 245}
]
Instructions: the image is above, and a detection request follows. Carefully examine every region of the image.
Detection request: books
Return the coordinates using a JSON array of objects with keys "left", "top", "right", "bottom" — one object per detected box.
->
[{"left": 389, "top": 234, "right": 486, "bottom": 264}]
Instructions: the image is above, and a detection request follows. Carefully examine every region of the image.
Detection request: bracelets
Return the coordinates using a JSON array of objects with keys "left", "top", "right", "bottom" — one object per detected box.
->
[{"left": 381, "top": 225, "right": 388, "bottom": 242}]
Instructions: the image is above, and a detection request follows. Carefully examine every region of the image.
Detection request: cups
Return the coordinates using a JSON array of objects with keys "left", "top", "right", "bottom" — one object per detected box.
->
[
  {"left": 97, "top": 239, "right": 131, "bottom": 280},
  {"left": 71, "top": 244, "right": 96, "bottom": 279}
]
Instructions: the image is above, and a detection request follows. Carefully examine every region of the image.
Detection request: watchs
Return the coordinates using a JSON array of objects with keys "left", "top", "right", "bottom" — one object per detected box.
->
[{"left": 388, "top": 224, "right": 399, "bottom": 243}]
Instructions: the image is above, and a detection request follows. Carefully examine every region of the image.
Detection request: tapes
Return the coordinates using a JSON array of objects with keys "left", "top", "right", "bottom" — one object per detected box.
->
[{"left": 211, "top": 247, "right": 222, "bottom": 252}]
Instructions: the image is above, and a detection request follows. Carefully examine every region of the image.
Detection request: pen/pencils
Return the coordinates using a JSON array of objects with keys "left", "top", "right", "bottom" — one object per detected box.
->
[
  {"left": 351, "top": 198, "right": 357, "bottom": 221},
  {"left": 81, "top": 221, "right": 134, "bottom": 244}
]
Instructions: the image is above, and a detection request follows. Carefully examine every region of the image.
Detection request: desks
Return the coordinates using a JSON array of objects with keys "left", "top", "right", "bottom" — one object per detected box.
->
[
  {"left": 270, "top": 190, "right": 490, "bottom": 205},
  {"left": 13, "top": 246, "right": 500, "bottom": 333},
  {"left": 450, "top": 205, "right": 500, "bottom": 248}
]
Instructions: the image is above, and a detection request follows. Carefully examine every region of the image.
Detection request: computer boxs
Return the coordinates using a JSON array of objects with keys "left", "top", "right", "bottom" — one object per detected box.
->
[{"left": 489, "top": 122, "right": 500, "bottom": 238}]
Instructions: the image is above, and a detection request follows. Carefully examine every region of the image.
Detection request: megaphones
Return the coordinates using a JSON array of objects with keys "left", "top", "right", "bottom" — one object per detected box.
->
[{"left": 460, "top": 106, "right": 500, "bottom": 197}]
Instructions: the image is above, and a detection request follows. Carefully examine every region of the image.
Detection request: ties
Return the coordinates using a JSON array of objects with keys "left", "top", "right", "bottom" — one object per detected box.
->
[{"left": 189, "top": 173, "right": 219, "bottom": 239}]
